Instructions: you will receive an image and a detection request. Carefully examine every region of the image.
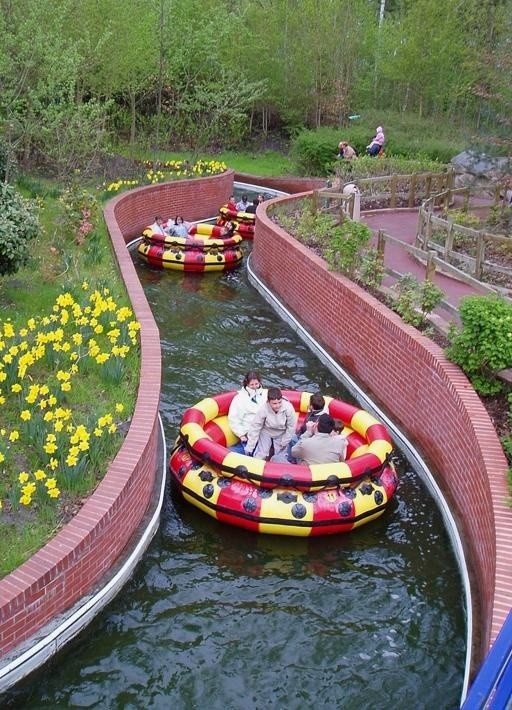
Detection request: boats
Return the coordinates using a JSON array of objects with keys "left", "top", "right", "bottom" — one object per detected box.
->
[
  {"left": 167, "top": 387, "right": 396, "bottom": 541},
  {"left": 139, "top": 221, "right": 242, "bottom": 274},
  {"left": 216, "top": 203, "right": 258, "bottom": 239},
  {"left": 169, "top": 482, "right": 394, "bottom": 574},
  {"left": 145, "top": 267, "right": 238, "bottom": 302}
]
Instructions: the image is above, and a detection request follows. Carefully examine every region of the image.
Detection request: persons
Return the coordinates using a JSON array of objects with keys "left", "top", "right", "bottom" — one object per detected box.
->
[
  {"left": 227, "top": 370, "right": 350, "bottom": 464},
  {"left": 337, "top": 126, "right": 384, "bottom": 160},
  {"left": 151, "top": 193, "right": 264, "bottom": 240}
]
